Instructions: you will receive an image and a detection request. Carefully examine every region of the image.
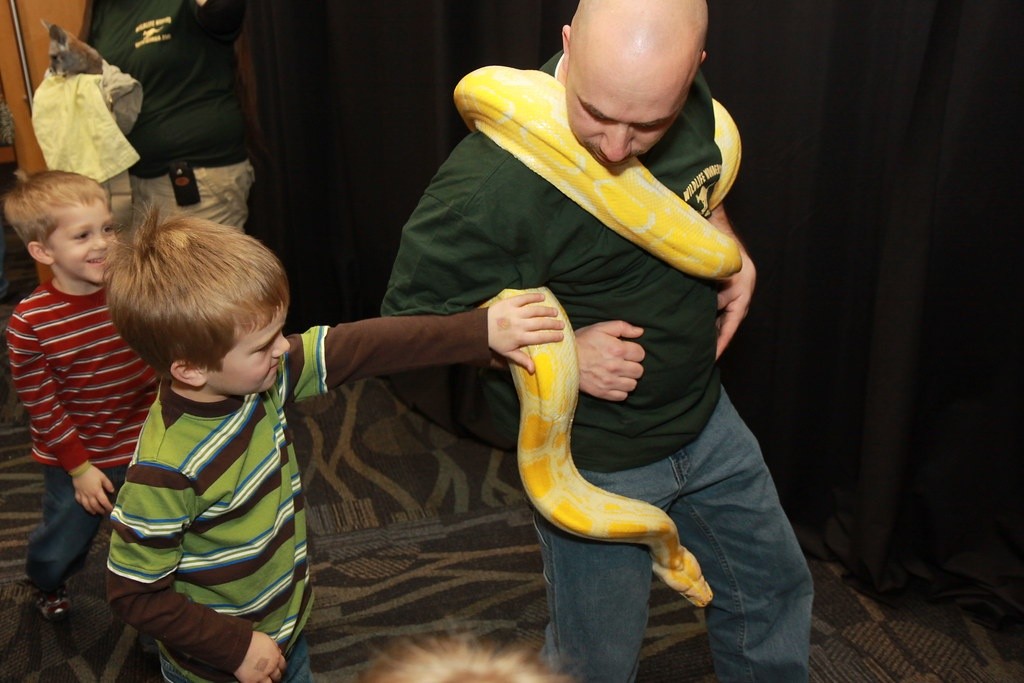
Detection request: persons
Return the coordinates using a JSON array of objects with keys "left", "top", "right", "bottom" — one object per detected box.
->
[
  {"left": 51, "top": 0, "right": 255, "bottom": 234},
  {"left": 2, "top": 169, "right": 162, "bottom": 620},
  {"left": 361, "top": 631, "right": 573, "bottom": 683},
  {"left": 380, "top": 1, "right": 815, "bottom": 683},
  {"left": 103, "top": 192, "right": 564, "bottom": 683},
  {"left": 0, "top": 216, "right": 18, "bottom": 305}
]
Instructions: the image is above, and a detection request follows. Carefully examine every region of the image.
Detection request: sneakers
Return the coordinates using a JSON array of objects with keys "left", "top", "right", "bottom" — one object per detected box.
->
[{"left": 36, "top": 584, "right": 73, "bottom": 622}]
